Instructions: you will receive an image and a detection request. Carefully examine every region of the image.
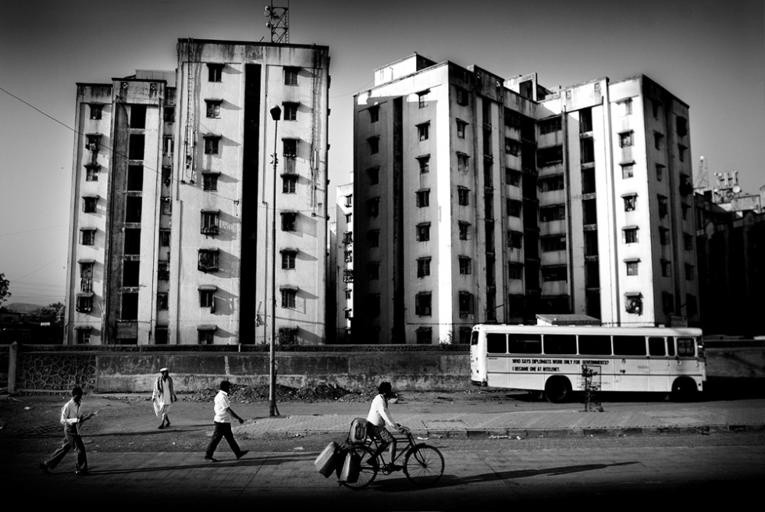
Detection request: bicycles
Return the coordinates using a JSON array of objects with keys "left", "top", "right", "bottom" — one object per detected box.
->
[{"left": 334, "top": 422, "right": 446, "bottom": 490}]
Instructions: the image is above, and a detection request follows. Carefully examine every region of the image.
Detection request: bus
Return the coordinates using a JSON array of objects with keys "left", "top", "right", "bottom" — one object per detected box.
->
[{"left": 466, "top": 320, "right": 708, "bottom": 403}]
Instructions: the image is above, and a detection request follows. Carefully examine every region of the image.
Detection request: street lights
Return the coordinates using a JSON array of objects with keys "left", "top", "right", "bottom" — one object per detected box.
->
[{"left": 267, "top": 103, "right": 283, "bottom": 417}]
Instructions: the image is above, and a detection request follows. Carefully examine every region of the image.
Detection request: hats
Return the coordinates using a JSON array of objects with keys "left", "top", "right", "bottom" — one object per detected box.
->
[
  {"left": 72, "top": 386, "right": 84, "bottom": 396},
  {"left": 160, "top": 367, "right": 168, "bottom": 371}
]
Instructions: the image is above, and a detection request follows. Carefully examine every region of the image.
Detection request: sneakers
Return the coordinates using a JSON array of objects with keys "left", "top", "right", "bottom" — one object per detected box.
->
[
  {"left": 203, "top": 456, "right": 215, "bottom": 462},
  {"left": 235, "top": 450, "right": 248, "bottom": 459},
  {"left": 158, "top": 422, "right": 170, "bottom": 429}
]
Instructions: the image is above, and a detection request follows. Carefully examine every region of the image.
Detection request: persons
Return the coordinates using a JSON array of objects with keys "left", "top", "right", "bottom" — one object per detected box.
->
[
  {"left": 365, "top": 381, "right": 408, "bottom": 472},
  {"left": 203, "top": 380, "right": 250, "bottom": 461},
  {"left": 39, "top": 386, "right": 95, "bottom": 478},
  {"left": 151, "top": 367, "right": 179, "bottom": 429}
]
